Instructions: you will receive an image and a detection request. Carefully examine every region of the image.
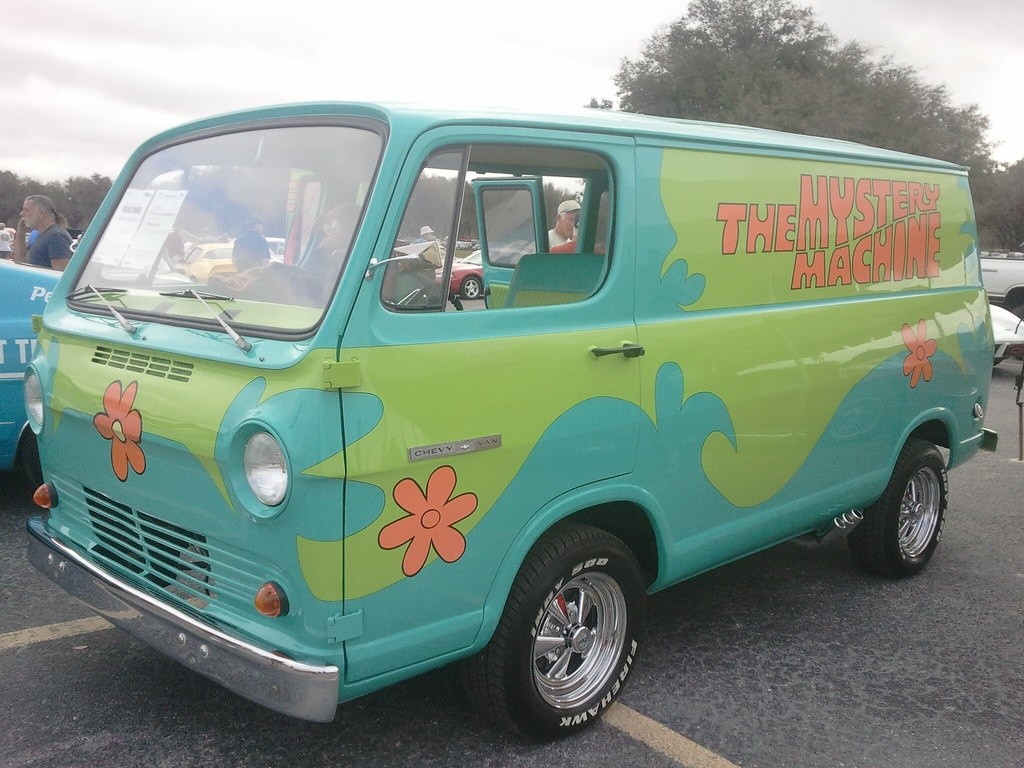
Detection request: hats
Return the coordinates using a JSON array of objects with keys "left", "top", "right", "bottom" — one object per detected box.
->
[
  {"left": 419, "top": 226, "right": 434, "bottom": 236},
  {"left": 557, "top": 199, "right": 581, "bottom": 214}
]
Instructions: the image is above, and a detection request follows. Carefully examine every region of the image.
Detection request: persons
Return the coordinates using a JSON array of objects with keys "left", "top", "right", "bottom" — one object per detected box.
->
[
  {"left": 551, "top": 209, "right": 606, "bottom": 255},
  {"left": 0, "top": 223, "right": 12, "bottom": 259},
  {"left": 14, "top": 194, "right": 74, "bottom": 271},
  {"left": 168, "top": 225, "right": 186, "bottom": 258},
  {"left": 548, "top": 198, "right": 581, "bottom": 254},
  {"left": 217, "top": 231, "right": 317, "bottom": 308},
  {"left": 412, "top": 225, "right": 435, "bottom": 244}
]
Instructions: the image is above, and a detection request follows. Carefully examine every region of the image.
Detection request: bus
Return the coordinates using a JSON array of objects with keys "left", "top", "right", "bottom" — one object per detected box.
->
[{"left": 22, "top": 99, "right": 1000, "bottom": 744}]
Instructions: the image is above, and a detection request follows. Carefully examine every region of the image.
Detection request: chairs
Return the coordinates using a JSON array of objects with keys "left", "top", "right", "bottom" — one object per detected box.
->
[{"left": 502, "top": 253, "right": 606, "bottom": 309}]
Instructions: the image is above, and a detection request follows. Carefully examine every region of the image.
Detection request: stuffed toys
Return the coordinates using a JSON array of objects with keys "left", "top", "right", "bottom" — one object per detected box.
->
[{"left": 316, "top": 205, "right": 361, "bottom": 267}]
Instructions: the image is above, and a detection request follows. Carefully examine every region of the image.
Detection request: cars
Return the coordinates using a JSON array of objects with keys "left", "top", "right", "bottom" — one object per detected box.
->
[
  {"left": 979, "top": 251, "right": 1024, "bottom": 367},
  {"left": 0, "top": 236, "right": 286, "bottom": 489},
  {"left": 434, "top": 239, "right": 529, "bottom": 301}
]
